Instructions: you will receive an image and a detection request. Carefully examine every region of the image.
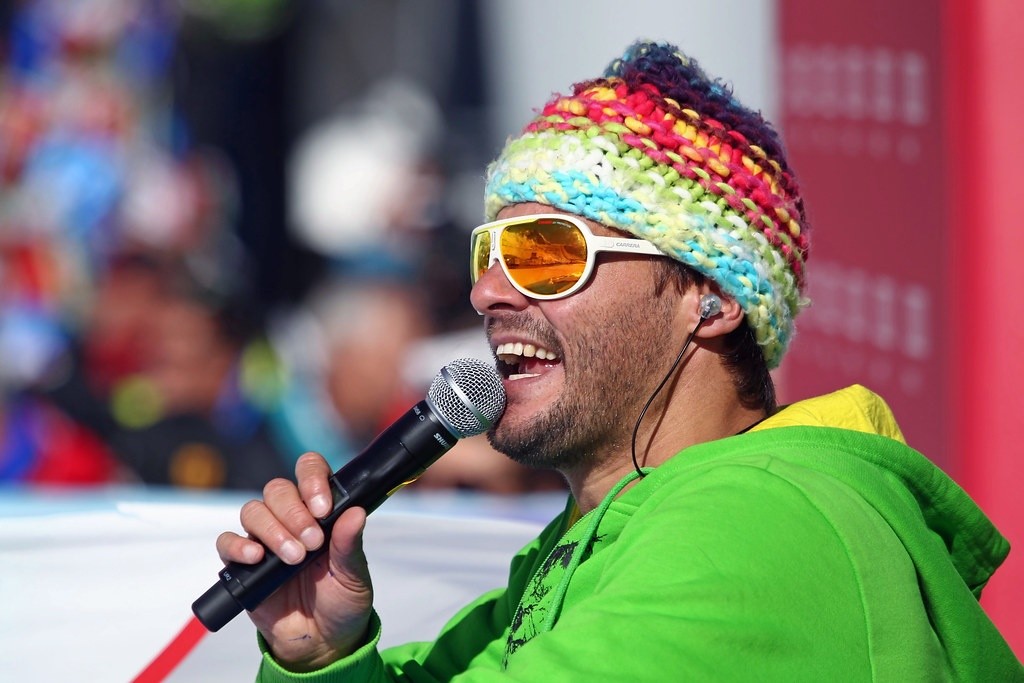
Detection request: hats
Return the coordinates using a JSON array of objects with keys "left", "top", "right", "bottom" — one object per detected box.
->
[{"left": 480, "top": 40, "right": 807, "bottom": 372}]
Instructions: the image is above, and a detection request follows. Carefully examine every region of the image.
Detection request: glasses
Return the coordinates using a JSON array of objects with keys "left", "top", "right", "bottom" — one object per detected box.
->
[{"left": 470, "top": 214, "right": 668, "bottom": 302}]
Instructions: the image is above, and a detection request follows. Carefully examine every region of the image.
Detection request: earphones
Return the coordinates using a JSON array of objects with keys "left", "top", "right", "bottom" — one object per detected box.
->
[{"left": 699, "top": 293, "right": 722, "bottom": 321}]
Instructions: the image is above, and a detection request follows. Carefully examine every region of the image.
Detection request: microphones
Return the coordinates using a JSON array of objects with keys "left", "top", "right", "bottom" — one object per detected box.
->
[{"left": 191, "top": 358, "right": 507, "bottom": 633}]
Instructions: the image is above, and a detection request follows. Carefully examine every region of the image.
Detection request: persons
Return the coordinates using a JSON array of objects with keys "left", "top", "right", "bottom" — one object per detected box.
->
[
  {"left": 216, "top": 41, "right": 1023, "bottom": 683},
  {"left": 0, "top": 0, "right": 564, "bottom": 496}
]
